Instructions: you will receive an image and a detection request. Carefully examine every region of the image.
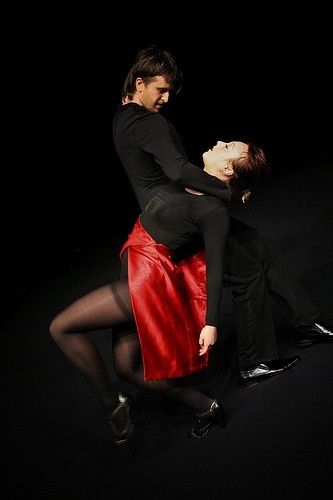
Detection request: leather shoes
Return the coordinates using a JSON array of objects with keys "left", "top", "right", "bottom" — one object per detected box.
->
[
  {"left": 295, "top": 321, "right": 333, "bottom": 348},
  {"left": 237, "top": 356, "right": 299, "bottom": 388}
]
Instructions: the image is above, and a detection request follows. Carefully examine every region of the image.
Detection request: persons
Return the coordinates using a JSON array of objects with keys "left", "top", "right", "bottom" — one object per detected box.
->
[
  {"left": 112, "top": 47, "right": 333, "bottom": 390},
  {"left": 49, "top": 140, "right": 270, "bottom": 445}
]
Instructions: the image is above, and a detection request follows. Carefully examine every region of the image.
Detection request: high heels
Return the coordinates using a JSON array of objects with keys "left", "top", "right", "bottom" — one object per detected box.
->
[
  {"left": 106, "top": 394, "right": 142, "bottom": 451},
  {"left": 187, "top": 400, "right": 224, "bottom": 439}
]
